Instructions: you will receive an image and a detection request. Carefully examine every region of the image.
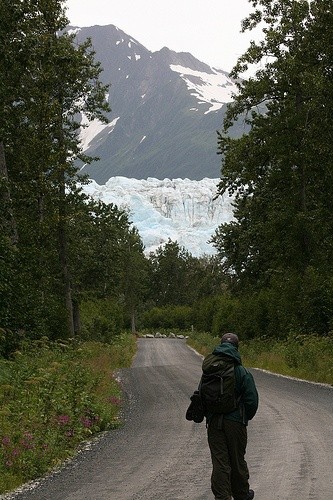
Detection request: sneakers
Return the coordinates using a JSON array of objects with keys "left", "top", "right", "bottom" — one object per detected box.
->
[
  {"left": 225, "top": 496, "right": 232, "bottom": 500},
  {"left": 245, "top": 490, "right": 255, "bottom": 500}
]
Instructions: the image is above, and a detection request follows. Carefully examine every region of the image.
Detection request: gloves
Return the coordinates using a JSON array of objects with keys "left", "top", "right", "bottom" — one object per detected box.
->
[{"left": 185, "top": 391, "right": 204, "bottom": 423}]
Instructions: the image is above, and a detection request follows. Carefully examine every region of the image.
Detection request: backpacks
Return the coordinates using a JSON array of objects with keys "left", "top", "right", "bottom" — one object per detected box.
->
[{"left": 198, "top": 353, "right": 242, "bottom": 415}]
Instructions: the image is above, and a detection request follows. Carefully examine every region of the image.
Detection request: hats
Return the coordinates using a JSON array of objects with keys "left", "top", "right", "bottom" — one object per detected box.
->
[{"left": 220, "top": 333, "right": 238, "bottom": 351}]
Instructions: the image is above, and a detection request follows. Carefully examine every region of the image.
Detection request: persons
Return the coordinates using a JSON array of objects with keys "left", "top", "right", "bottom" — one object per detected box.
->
[{"left": 197, "top": 333, "right": 259, "bottom": 500}]
